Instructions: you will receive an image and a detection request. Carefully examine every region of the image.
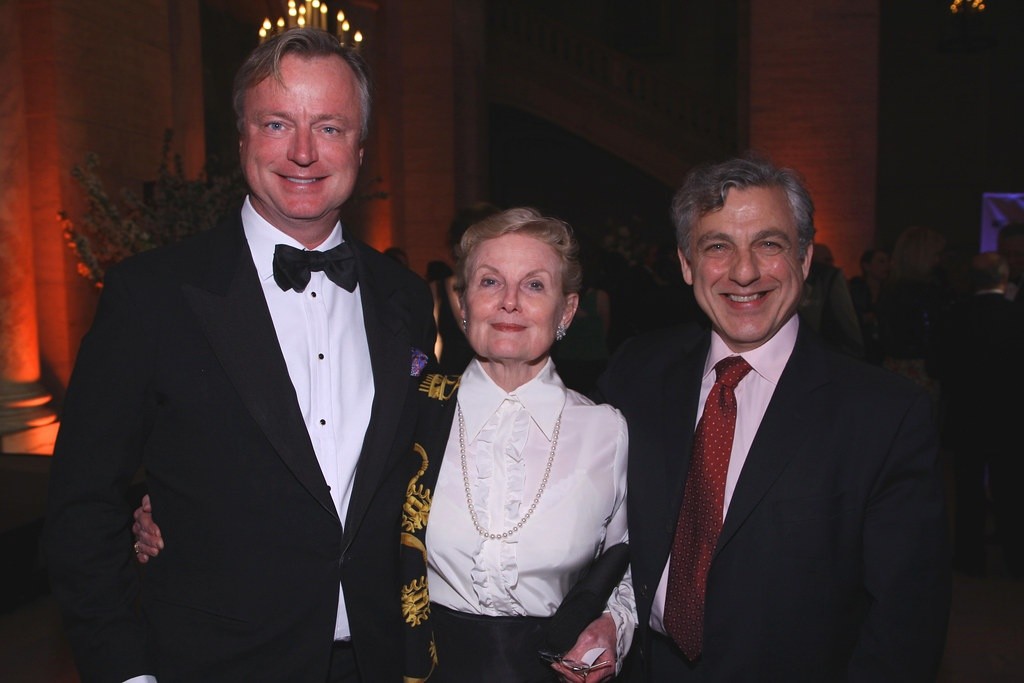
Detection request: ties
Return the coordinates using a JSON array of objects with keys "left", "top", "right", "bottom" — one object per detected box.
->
[{"left": 664, "top": 356, "right": 752, "bottom": 665}]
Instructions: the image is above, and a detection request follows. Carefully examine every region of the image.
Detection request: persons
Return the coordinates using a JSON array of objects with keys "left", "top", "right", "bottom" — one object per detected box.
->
[
  {"left": 823, "top": 215, "right": 1021, "bottom": 593},
  {"left": 632, "top": 160, "right": 939, "bottom": 680},
  {"left": 363, "top": 173, "right": 663, "bottom": 398},
  {"left": 44, "top": 30, "right": 435, "bottom": 683},
  {"left": 132, "top": 205, "right": 640, "bottom": 681}
]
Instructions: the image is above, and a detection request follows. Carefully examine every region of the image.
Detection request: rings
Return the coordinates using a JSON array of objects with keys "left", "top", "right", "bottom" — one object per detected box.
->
[{"left": 134, "top": 540, "right": 142, "bottom": 553}]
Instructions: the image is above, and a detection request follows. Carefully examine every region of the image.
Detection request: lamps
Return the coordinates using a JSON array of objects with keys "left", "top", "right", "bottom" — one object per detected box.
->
[{"left": 259, "top": 0, "right": 364, "bottom": 49}]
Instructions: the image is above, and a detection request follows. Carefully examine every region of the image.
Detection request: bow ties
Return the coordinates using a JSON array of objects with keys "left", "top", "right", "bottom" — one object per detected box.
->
[{"left": 272, "top": 241, "right": 359, "bottom": 295}]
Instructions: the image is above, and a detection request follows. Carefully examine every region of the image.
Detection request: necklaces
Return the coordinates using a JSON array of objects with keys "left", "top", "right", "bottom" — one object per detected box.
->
[{"left": 455, "top": 386, "right": 567, "bottom": 542}]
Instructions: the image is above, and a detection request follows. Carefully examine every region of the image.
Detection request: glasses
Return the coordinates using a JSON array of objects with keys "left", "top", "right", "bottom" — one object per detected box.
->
[{"left": 539, "top": 646, "right": 612, "bottom": 683}]
